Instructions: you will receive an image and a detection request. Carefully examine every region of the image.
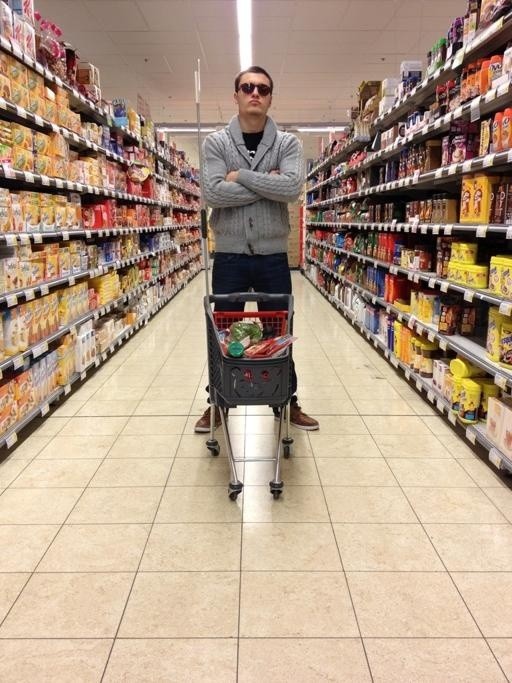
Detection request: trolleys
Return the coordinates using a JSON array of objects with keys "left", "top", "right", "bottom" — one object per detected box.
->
[{"left": 201, "top": 288, "right": 303, "bottom": 501}]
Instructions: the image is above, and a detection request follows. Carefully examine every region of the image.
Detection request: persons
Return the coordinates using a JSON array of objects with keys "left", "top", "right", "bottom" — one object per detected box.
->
[{"left": 191, "top": 64, "right": 320, "bottom": 435}]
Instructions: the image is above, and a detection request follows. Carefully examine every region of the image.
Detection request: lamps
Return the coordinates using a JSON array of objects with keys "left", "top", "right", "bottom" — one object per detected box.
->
[{"left": 234, "top": 1, "right": 255, "bottom": 75}]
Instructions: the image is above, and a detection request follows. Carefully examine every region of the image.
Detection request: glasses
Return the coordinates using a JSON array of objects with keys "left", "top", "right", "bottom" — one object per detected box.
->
[{"left": 237, "top": 83, "right": 271, "bottom": 96}]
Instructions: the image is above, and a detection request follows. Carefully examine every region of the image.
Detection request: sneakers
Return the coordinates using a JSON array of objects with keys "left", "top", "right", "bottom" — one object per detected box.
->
[
  {"left": 274, "top": 402, "right": 319, "bottom": 431},
  {"left": 195, "top": 406, "right": 228, "bottom": 433}
]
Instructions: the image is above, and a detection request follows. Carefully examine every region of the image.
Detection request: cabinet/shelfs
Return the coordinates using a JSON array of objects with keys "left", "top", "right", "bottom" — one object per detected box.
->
[
  {"left": 1, "top": 1, "right": 205, "bottom": 460},
  {"left": 301, "top": 1, "right": 512, "bottom": 477}
]
